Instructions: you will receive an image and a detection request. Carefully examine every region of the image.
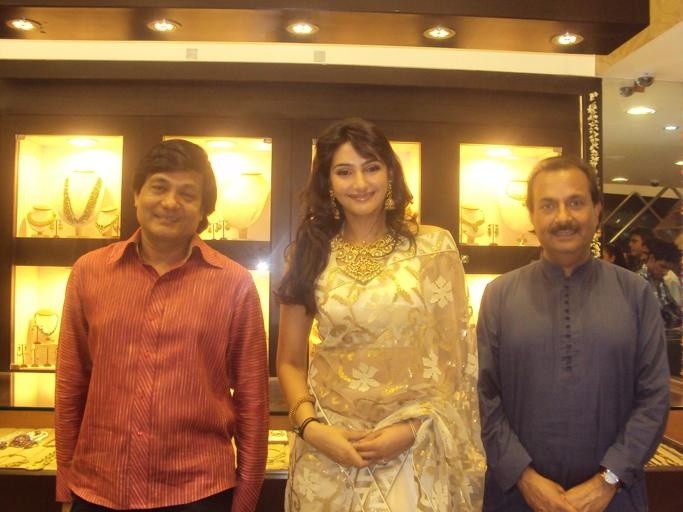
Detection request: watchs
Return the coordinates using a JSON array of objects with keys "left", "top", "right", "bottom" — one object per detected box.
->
[{"left": 601, "top": 467, "right": 620, "bottom": 490}]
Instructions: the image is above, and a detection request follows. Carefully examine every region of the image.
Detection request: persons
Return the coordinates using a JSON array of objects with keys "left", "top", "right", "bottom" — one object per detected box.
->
[
  {"left": 276, "top": 115, "right": 470, "bottom": 512},
  {"left": 603, "top": 228, "right": 683, "bottom": 328},
  {"left": 53, "top": 138, "right": 269, "bottom": 511},
  {"left": 477, "top": 156, "right": 670, "bottom": 512}
]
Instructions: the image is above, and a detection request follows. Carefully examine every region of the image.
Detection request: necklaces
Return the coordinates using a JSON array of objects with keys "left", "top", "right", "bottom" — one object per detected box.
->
[{"left": 338, "top": 209, "right": 385, "bottom": 244}]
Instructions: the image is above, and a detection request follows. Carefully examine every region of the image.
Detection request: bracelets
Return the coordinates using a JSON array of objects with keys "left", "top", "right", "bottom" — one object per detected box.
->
[
  {"left": 287, "top": 395, "right": 322, "bottom": 439},
  {"left": 406, "top": 417, "right": 421, "bottom": 444}
]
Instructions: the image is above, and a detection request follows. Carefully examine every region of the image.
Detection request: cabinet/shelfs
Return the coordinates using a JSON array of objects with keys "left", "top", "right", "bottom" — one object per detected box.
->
[
  {"left": 0, "top": 79, "right": 293, "bottom": 414},
  {"left": 294, "top": 81, "right": 595, "bottom": 379},
  {"left": 1, "top": 374, "right": 683, "bottom": 511}
]
